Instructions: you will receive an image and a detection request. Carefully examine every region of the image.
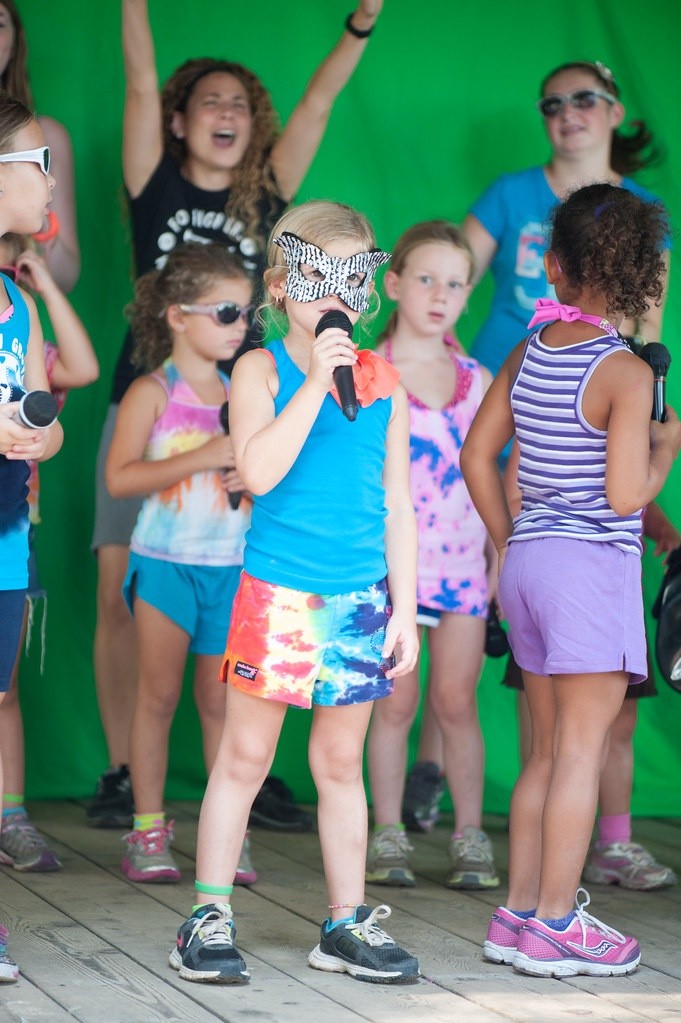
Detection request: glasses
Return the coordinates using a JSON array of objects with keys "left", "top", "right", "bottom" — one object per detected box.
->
[
  {"left": 536, "top": 89, "right": 617, "bottom": 118},
  {"left": 0, "top": 146, "right": 51, "bottom": 176},
  {"left": 158, "top": 302, "right": 257, "bottom": 328}
]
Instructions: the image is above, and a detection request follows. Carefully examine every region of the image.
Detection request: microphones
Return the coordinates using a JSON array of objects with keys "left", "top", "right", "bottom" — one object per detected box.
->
[
  {"left": 219, "top": 401, "right": 243, "bottom": 510},
  {"left": 640, "top": 343, "right": 672, "bottom": 423},
  {"left": 315, "top": 311, "right": 358, "bottom": 422},
  {"left": 11, "top": 390, "right": 58, "bottom": 429}
]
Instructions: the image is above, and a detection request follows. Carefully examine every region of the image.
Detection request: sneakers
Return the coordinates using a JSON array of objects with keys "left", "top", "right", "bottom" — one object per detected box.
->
[
  {"left": 365, "top": 824, "right": 417, "bottom": 886},
  {"left": 85, "top": 764, "right": 135, "bottom": 829},
  {"left": 122, "top": 818, "right": 181, "bottom": 883},
  {"left": 0, "top": 811, "right": 63, "bottom": 872},
  {"left": 581, "top": 841, "right": 678, "bottom": 891},
  {"left": 0, "top": 925, "right": 19, "bottom": 982},
  {"left": 232, "top": 830, "right": 257, "bottom": 885},
  {"left": 307, "top": 904, "right": 422, "bottom": 983},
  {"left": 483, "top": 907, "right": 527, "bottom": 966},
  {"left": 446, "top": 826, "right": 500, "bottom": 890},
  {"left": 512, "top": 887, "right": 641, "bottom": 978},
  {"left": 169, "top": 902, "right": 251, "bottom": 985},
  {"left": 249, "top": 776, "right": 310, "bottom": 832},
  {"left": 404, "top": 759, "right": 445, "bottom": 833}
]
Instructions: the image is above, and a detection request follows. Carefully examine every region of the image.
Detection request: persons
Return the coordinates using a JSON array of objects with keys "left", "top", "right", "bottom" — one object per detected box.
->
[
  {"left": 87, "top": 0, "right": 382, "bottom": 833},
  {"left": 400, "top": 62, "right": 681, "bottom": 890},
  {"left": 0, "top": 0, "right": 100, "bottom": 984},
  {"left": 459, "top": 185, "right": 680, "bottom": 978},
  {"left": 367, "top": 221, "right": 501, "bottom": 886},
  {"left": 167, "top": 202, "right": 422, "bottom": 985},
  {"left": 123, "top": 243, "right": 258, "bottom": 882}
]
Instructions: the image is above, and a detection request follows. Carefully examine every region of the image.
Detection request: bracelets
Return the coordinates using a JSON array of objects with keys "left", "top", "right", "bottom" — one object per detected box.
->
[{"left": 344, "top": 12, "right": 375, "bottom": 37}]
